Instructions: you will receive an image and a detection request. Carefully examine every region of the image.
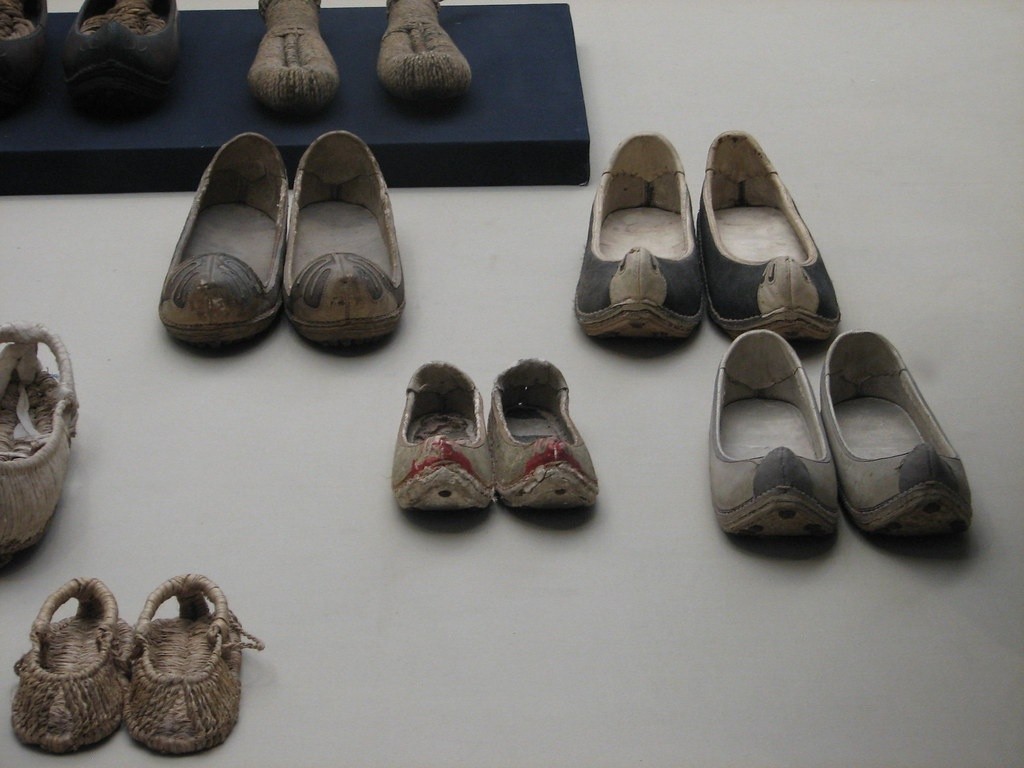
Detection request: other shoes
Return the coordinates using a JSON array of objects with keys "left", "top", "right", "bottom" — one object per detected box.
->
[
  {"left": 282, "top": 130, "right": 406, "bottom": 344},
  {"left": 698, "top": 129, "right": 840, "bottom": 343},
  {"left": 820, "top": 330, "right": 972, "bottom": 534},
  {"left": 159, "top": 132, "right": 288, "bottom": 350},
  {"left": 0, "top": 322, "right": 78, "bottom": 556},
  {"left": 125, "top": 574, "right": 265, "bottom": 754},
  {"left": 489, "top": 358, "right": 598, "bottom": 509},
  {"left": 392, "top": 358, "right": 492, "bottom": 510},
  {"left": 10, "top": 579, "right": 131, "bottom": 753},
  {"left": 0, "top": 0, "right": 47, "bottom": 84},
  {"left": 709, "top": 329, "right": 838, "bottom": 537},
  {"left": 575, "top": 132, "right": 704, "bottom": 339},
  {"left": 63, "top": 0, "right": 178, "bottom": 102}
]
